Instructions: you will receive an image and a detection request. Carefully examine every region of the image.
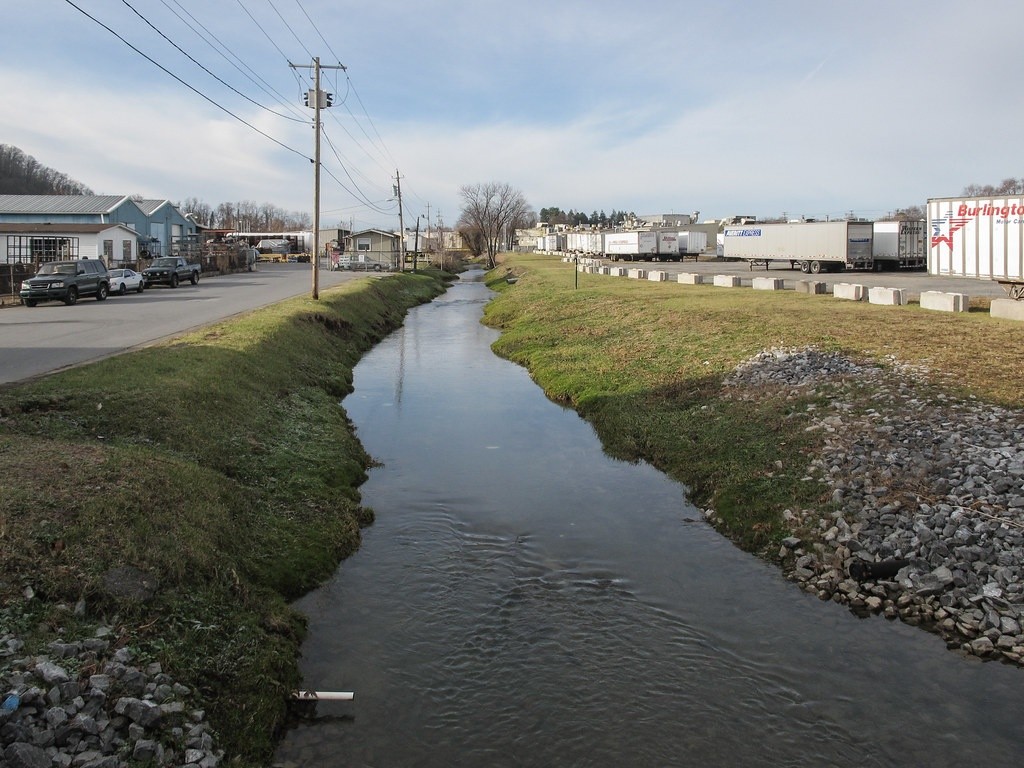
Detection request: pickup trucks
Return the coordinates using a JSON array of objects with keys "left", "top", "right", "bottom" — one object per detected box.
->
[{"left": 141, "top": 256, "right": 201, "bottom": 288}]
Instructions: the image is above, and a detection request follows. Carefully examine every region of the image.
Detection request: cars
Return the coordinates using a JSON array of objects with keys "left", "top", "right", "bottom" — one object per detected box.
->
[
  {"left": 330, "top": 254, "right": 390, "bottom": 271},
  {"left": 107, "top": 269, "right": 145, "bottom": 295}
]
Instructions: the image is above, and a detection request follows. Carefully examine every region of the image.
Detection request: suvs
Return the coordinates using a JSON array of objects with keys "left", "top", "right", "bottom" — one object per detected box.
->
[{"left": 19, "top": 259, "right": 111, "bottom": 307}]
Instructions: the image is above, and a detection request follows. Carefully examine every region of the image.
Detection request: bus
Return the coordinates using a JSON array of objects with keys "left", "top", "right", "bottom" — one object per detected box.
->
[{"left": 226, "top": 232, "right": 310, "bottom": 254}]
